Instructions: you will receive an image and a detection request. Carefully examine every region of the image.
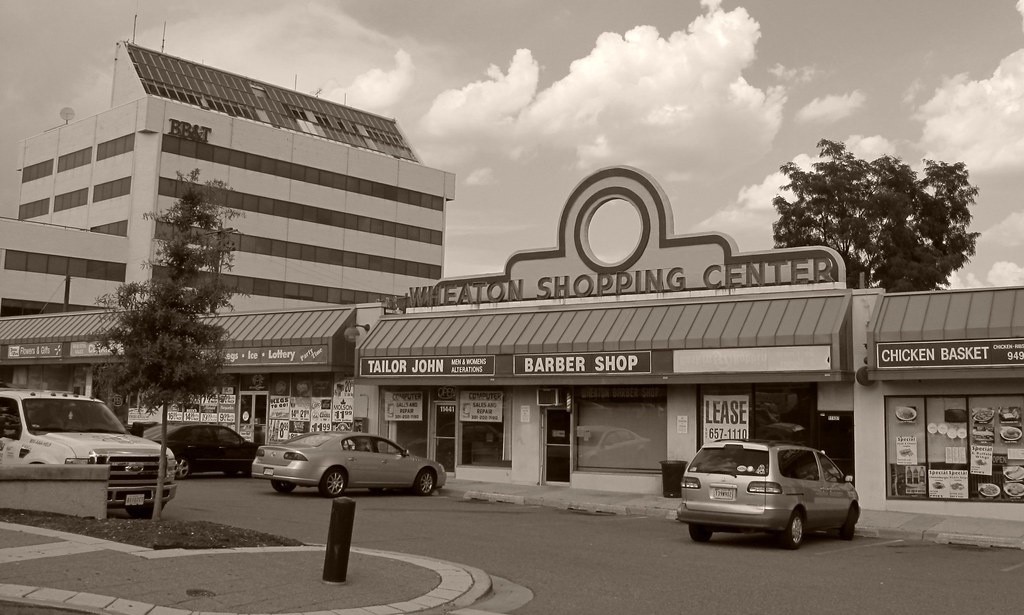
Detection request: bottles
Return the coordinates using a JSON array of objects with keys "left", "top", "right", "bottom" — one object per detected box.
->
[{"left": 907, "top": 466, "right": 925, "bottom": 484}]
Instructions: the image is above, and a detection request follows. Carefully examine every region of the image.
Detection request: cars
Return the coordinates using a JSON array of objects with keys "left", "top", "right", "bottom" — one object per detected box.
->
[
  {"left": 676, "top": 438, "right": 861, "bottom": 549},
  {"left": 142, "top": 423, "right": 261, "bottom": 481},
  {"left": 251, "top": 430, "right": 446, "bottom": 499}
]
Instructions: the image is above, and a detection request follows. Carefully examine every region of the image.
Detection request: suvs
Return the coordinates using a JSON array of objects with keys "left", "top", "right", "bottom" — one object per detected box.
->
[{"left": 0, "top": 382, "right": 179, "bottom": 519}]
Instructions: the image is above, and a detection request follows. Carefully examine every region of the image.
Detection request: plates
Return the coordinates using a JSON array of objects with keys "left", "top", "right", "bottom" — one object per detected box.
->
[
  {"left": 979, "top": 484, "right": 1000, "bottom": 497},
  {"left": 973, "top": 410, "right": 994, "bottom": 422},
  {"left": 927, "top": 423, "right": 967, "bottom": 439},
  {"left": 1000, "top": 427, "right": 1022, "bottom": 440},
  {"left": 895, "top": 407, "right": 917, "bottom": 421},
  {"left": 1002, "top": 466, "right": 1024, "bottom": 497}
]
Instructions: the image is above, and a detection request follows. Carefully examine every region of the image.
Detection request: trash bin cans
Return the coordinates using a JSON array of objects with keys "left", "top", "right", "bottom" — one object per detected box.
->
[{"left": 659, "top": 460, "right": 687, "bottom": 498}]
